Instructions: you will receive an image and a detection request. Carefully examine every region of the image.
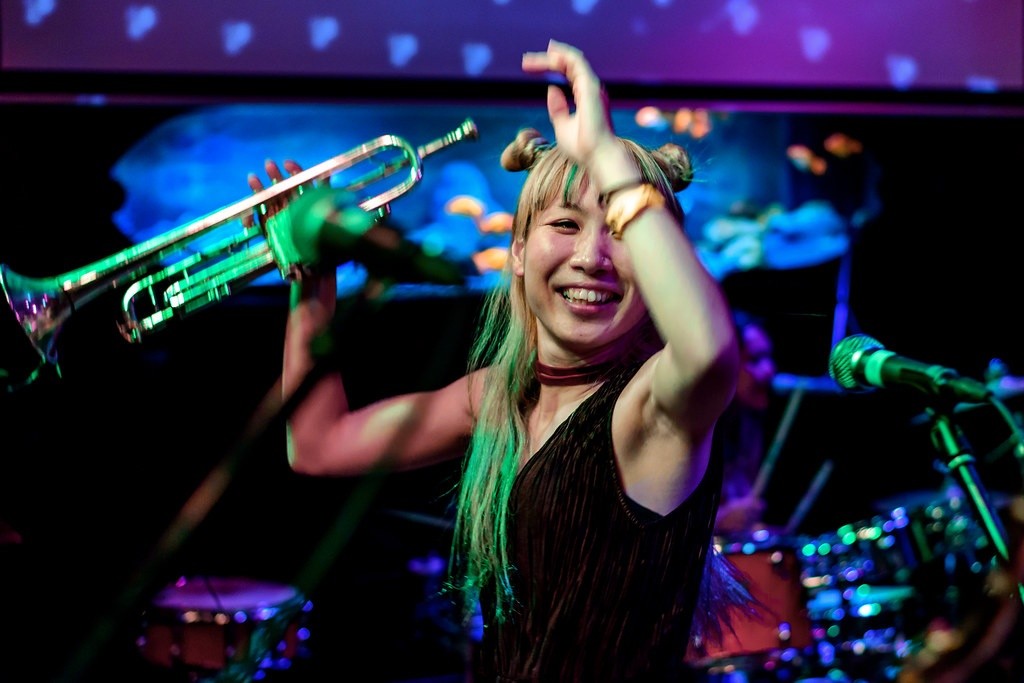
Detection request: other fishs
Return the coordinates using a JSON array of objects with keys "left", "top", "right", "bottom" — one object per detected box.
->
[
  {"left": 635, "top": 105, "right": 710, "bottom": 140},
  {"left": 448, "top": 192, "right": 516, "bottom": 269},
  {"left": 699, "top": 201, "right": 847, "bottom": 270},
  {"left": 786, "top": 135, "right": 863, "bottom": 177}
]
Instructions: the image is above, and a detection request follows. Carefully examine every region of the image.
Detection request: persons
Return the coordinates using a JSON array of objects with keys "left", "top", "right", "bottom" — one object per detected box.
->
[
  {"left": 239, "top": 37, "right": 740, "bottom": 683},
  {"left": 922, "top": 497, "right": 1024, "bottom": 683},
  {"left": 710, "top": 312, "right": 789, "bottom": 543}
]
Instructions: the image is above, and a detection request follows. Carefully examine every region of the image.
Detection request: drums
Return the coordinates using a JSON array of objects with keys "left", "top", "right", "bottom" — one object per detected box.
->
[
  {"left": 683, "top": 540, "right": 814, "bottom": 671},
  {"left": 132, "top": 578, "right": 304, "bottom": 677}
]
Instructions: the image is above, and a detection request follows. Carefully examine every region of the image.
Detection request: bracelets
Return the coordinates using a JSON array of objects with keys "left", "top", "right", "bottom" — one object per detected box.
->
[{"left": 598, "top": 177, "right": 665, "bottom": 240}]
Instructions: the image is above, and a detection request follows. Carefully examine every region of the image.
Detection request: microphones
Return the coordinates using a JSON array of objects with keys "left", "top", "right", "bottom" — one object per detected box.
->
[
  {"left": 290, "top": 190, "right": 473, "bottom": 288},
  {"left": 829, "top": 334, "right": 993, "bottom": 404},
  {"left": 774, "top": 373, "right": 842, "bottom": 394}
]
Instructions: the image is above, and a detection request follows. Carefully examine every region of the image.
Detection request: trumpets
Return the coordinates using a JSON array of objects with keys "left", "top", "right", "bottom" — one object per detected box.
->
[{"left": 2, "top": 119, "right": 482, "bottom": 360}]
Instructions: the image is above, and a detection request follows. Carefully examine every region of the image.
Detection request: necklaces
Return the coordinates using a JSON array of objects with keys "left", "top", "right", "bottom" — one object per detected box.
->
[{"left": 535, "top": 359, "right": 620, "bottom": 386}]
